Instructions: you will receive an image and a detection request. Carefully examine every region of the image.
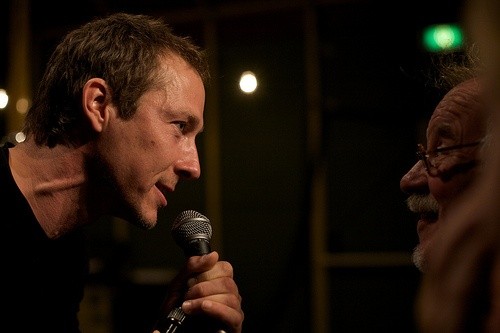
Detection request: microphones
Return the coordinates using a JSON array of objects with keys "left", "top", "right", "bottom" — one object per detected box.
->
[
  {"left": 163, "top": 307, "right": 186, "bottom": 333},
  {"left": 172, "top": 210, "right": 231, "bottom": 333}
]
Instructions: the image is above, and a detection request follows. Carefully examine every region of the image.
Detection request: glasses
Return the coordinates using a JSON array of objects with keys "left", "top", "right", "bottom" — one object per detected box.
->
[{"left": 416, "top": 141, "right": 483, "bottom": 173}]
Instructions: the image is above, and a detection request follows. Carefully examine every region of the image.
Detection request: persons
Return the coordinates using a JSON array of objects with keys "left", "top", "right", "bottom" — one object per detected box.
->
[
  {"left": 398, "top": 42, "right": 500, "bottom": 333},
  {"left": 0, "top": 13, "right": 245, "bottom": 333}
]
